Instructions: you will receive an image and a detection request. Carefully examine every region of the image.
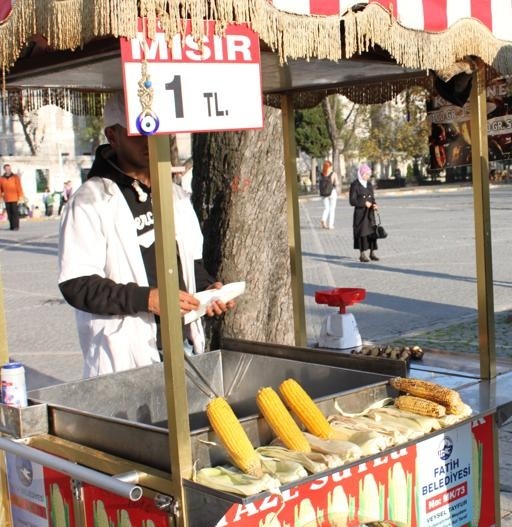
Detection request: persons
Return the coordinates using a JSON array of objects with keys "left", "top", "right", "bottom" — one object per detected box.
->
[
  {"left": 57, "top": 90, "right": 238, "bottom": 380},
  {"left": 0, "top": 164, "right": 25, "bottom": 232},
  {"left": 348, "top": 164, "right": 380, "bottom": 263},
  {"left": 315, "top": 158, "right": 343, "bottom": 229},
  {"left": 19, "top": 177, "right": 75, "bottom": 218}
]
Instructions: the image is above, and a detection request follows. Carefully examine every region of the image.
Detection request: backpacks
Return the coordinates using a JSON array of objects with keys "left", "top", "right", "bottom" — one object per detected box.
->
[{"left": 318, "top": 172, "right": 335, "bottom": 196}]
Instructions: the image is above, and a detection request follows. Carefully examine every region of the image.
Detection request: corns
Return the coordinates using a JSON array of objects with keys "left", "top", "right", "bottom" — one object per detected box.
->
[
  {"left": 393, "top": 396, "right": 446, "bottom": 418},
  {"left": 278, "top": 378, "right": 334, "bottom": 440},
  {"left": 206, "top": 398, "right": 263, "bottom": 477},
  {"left": 390, "top": 378, "right": 460, "bottom": 405},
  {"left": 255, "top": 387, "right": 312, "bottom": 456},
  {"left": 446, "top": 402, "right": 464, "bottom": 415}
]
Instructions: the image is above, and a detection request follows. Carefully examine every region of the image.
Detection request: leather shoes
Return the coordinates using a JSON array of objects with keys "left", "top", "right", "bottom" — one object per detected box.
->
[
  {"left": 371, "top": 256, "right": 378, "bottom": 260},
  {"left": 360, "top": 257, "right": 368, "bottom": 262}
]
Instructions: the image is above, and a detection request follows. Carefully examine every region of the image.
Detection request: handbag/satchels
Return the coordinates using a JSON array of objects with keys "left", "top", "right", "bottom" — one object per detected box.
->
[
  {"left": 374, "top": 225, "right": 387, "bottom": 238},
  {"left": 17, "top": 204, "right": 28, "bottom": 216}
]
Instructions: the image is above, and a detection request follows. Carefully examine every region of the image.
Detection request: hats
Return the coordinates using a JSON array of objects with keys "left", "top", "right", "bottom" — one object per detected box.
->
[
  {"left": 102, "top": 90, "right": 127, "bottom": 131},
  {"left": 360, "top": 163, "right": 372, "bottom": 177}
]
